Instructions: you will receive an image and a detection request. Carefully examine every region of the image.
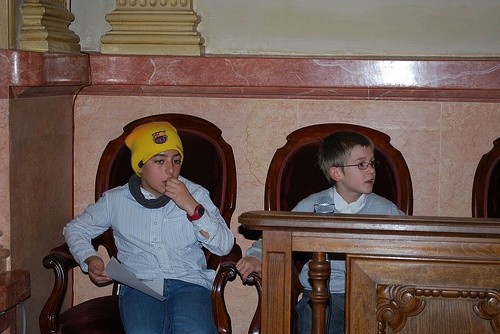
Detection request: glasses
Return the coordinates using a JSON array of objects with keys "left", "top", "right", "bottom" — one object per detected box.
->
[{"left": 332, "top": 161, "right": 380, "bottom": 170}]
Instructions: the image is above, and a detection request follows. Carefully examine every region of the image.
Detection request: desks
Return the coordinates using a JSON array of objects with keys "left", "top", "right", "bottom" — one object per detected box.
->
[{"left": 238, "top": 211, "right": 500, "bottom": 334}]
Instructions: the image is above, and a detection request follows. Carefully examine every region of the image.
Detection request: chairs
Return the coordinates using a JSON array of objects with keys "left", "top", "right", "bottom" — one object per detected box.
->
[
  {"left": 244, "top": 122, "right": 413, "bottom": 334},
  {"left": 471, "top": 137, "right": 500, "bottom": 218},
  {"left": 38, "top": 113, "right": 242, "bottom": 334}
]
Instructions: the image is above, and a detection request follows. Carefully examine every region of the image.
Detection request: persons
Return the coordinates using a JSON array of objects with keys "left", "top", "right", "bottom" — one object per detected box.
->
[
  {"left": 234, "top": 128, "right": 410, "bottom": 334},
  {"left": 60, "top": 121, "right": 234, "bottom": 334}
]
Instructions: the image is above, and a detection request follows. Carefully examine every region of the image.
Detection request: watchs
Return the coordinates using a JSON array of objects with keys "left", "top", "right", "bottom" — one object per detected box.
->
[{"left": 186, "top": 204, "right": 205, "bottom": 222}]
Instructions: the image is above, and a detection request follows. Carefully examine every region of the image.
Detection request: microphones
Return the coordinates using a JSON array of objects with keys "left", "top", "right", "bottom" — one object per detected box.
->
[{"left": 314, "top": 195, "right": 335, "bottom": 214}]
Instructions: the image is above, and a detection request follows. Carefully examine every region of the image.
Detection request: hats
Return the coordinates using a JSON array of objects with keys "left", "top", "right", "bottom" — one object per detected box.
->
[{"left": 125, "top": 121, "right": 184, "bottom": 177}]
